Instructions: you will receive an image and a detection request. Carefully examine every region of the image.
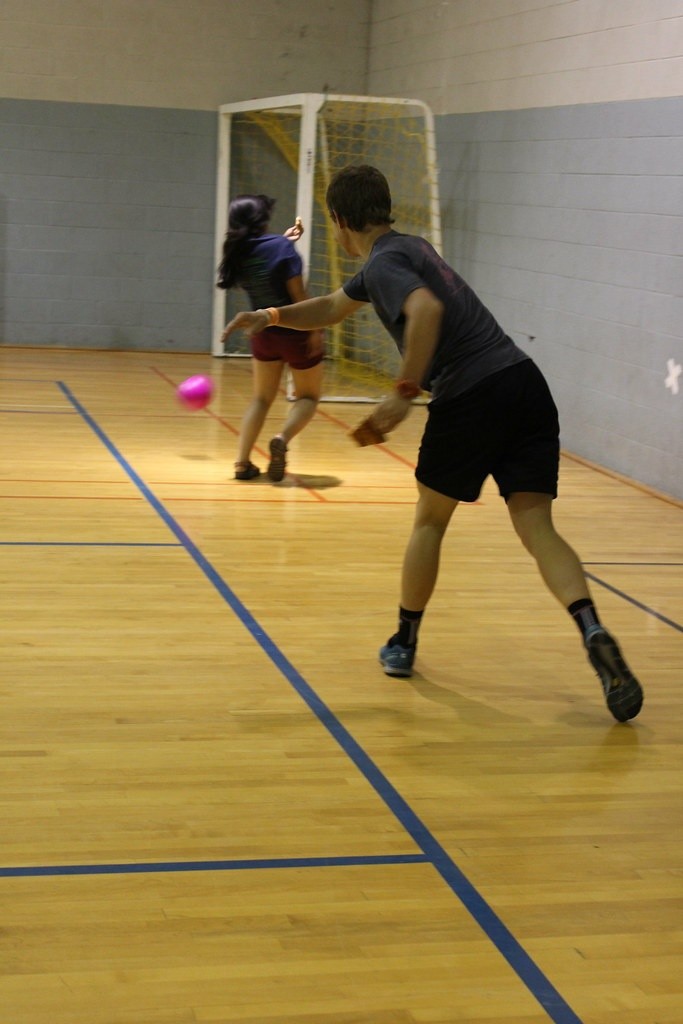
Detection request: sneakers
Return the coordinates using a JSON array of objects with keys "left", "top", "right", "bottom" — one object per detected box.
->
[
  {"left": 585, "top": 623, "right": 644, "bottom": 722},
  {"left": 235, "top": 461, "right": 261, "bottom": 480},
  {"left": 267, "top": 436, "right": 287, "bottom": 482},
  {"left": 379, "top": 635, "right": 417, "bottom": 675}
]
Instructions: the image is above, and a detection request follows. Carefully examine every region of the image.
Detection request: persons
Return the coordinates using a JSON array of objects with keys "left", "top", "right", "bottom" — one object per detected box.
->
[
  {"left": 221, "top": 166, "right": 644, "bottom": 722},
  {"left": 217, "top": 194, "right": 325, "bottom": 483}
]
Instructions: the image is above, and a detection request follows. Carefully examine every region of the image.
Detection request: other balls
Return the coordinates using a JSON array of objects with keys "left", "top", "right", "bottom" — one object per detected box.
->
[{"left": 176, "top": 373, "right": 215, "bottom": 411}]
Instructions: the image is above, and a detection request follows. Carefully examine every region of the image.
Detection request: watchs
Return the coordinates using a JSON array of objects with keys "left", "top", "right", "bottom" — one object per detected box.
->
[{"left": 396, "top": 379, "right": 424, "bottom": 400}]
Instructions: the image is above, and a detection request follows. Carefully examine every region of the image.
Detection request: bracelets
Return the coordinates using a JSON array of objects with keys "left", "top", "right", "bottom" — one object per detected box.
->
[{"left": 257, "top": 307, "right": 279, "bottom": 326}]
